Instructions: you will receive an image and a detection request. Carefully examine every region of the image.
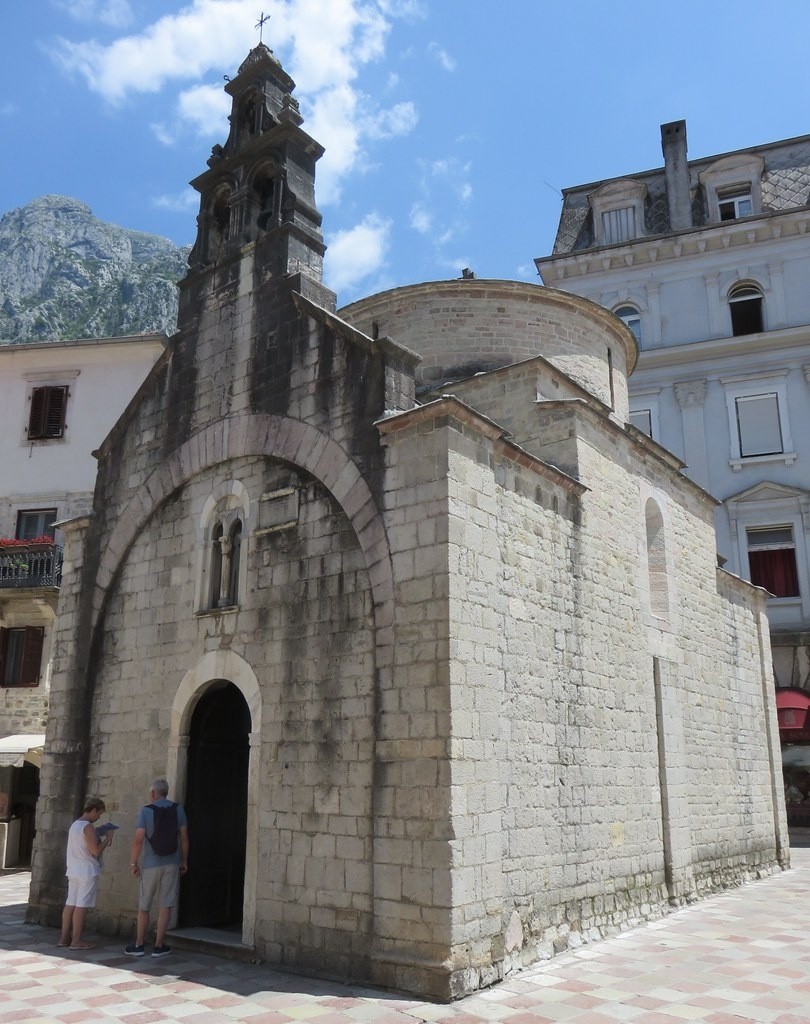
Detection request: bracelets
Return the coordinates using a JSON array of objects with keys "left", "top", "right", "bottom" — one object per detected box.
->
[{"left": 131, "top": 862, "right": 137, "bottom": 866}]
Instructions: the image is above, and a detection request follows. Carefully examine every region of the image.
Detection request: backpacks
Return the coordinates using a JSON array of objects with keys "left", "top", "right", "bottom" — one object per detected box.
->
[{"left": 145, "top": 801, "right": 179, "bottom": 856}]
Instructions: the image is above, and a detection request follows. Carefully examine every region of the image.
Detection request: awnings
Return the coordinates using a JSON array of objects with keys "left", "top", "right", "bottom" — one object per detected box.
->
[{"left": 0, "top": 732, "right": 46, "bottom": 769}]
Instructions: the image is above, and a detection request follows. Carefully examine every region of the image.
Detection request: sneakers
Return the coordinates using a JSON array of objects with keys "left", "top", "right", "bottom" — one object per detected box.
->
[
  {"left": 124, "top": 945, "right": 145, "bottom": 956},
  {"left": 151, "top": 944, "right": 171, "bottom": 957}
]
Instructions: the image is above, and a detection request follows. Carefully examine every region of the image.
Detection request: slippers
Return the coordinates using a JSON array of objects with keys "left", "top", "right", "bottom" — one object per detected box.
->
[
  {"left": 68, "top": 941, "right": 95, "bottom": 949},
  {"left": 58, "top": 940, "right": 70, "bottom": 946}
]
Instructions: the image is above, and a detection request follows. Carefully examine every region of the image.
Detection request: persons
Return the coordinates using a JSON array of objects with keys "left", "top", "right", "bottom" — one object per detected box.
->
[
  {"left": 125, "top": 779, "right": 189, "bottom": 958},
  {"left": 57, "top": 797, "right": 114, "bottom": 950}
]
